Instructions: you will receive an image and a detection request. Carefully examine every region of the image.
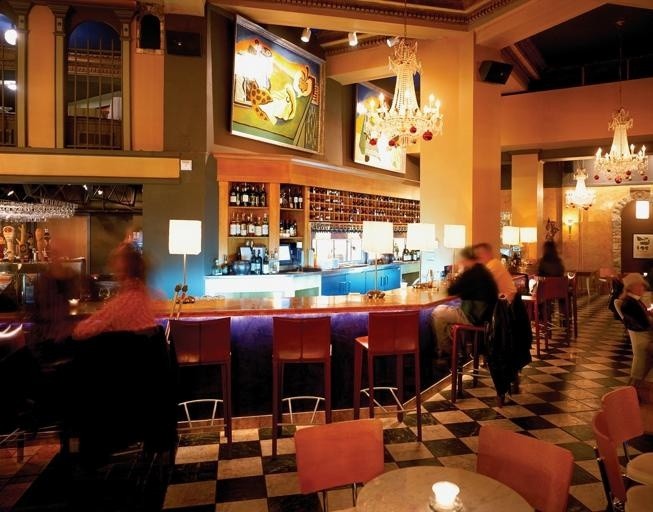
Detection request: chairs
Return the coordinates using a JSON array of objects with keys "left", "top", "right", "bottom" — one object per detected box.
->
[
  {"left": 451, "top": 324, "right": 518, "bottom": 408},
  {"left": 519, "top": 277, "right": 549, "bottom": 359},
  {"left": 352, "top": 310, "right": 422, "bottom": 443},
  {"left": 168, "top": 316, "right": 232, "bottom": 459},
  {"left": 592, "top": 410, "right": 653, "bottom": 512},
  {"left": 476, "top": 425, "right": 574, "bottom": 512},
  {"left": 294, "top": 418, "right": 383, "bottom": 512},
  {"left": 271, "top": 316, "right": 331, "bottom": 462},
  {"left": 569, "top": 272, "right": 577, "bottom": 339},
  {"left": 544, "top": 276, "right": 570, "bottom": 339},
  {"left": 602, "top": 386, "right": 653, "bottom": 486}
]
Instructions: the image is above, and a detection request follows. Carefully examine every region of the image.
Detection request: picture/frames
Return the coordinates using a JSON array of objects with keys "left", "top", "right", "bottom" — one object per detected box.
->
[
  {"left": 228, "top": 13, "right": 327, "bottom": 156},
  {"left": 352, "top": 81, "right": 407, "bottom": 174},
  {"left": 34, "top": 212, "right": 91, "bottom": 275}
]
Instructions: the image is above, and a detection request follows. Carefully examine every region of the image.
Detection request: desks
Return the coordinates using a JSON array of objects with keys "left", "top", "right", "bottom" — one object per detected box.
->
[{"left": 356, "top": 465, "right": 537, "bottom": 512}]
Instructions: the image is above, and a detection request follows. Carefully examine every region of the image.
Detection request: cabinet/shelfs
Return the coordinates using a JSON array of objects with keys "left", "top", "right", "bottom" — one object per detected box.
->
[
  {"left": 226, "top": 181, "right": 275, "bottom": 257},
  {"left": 277, "top": 184, "right": 308, "bottom": 250},
  {"left": 308, "top": 187, "right": 421, "bottom": 238}
]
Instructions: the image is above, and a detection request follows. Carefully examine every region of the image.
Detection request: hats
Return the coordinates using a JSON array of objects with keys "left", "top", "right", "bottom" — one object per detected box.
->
[{"left": 622, "top": 272, "right": 648, "bottom": 290}]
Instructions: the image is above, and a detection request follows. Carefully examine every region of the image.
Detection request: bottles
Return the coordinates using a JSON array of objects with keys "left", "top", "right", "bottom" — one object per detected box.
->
[
  {"left": 256, "top": 249, "right": 262, "bottom": 274},
  {"left": 310, "top": 187, "right": 420, "bottom": 224},
  {"left": 271, "top": 248, "right": 280, "bottom": 274},
  {"left": 250, "top": 250, "right": 256, "bottom": 274},
  {"left": 280, "top": 185, "right": 304, "bottom": 210},
  {"left": 221, "top": 255, "right": 229, "bottom": 275},
  {"left": 211, "top": 259, "right": 222, "bottom": 276},
  {"left": 262, "top": 248, "right": 269, "bottom": 274},
  {"left": 229, "top": 182, "right": 267, "bottom": 207},
  {"left": 279, "top": 217, "right": 298, "bottom": 237},
  {"left": 229, "top": 211, "right": 269, "bottom": 237}
]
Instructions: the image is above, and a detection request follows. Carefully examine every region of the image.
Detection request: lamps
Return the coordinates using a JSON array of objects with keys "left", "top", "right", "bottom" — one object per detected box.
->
[
  {"left": 564, "top": 161, "right": 598, "bottom": 212},
  {"left": 501, "top": 226, "right": 520, "bottom": 258},
  {"left": 425, "top": 478, "right": 464, "bottom": 512},
  {"left": 168, "top": 219, "right": 202, "bottom": 304},
  {"left": 406, "top": 223, "right": 435, "bottom": 289},
  {"left": 519, "top": 227, "right": 538, "bottom": 243},
  {"left": 443, "top": 225, "right": 466, "bottom": 264},
  {"left": 386, "top": 37, "right": 400, "bottom": 47},
  {"left": 362, "top": 221, "right": 394, "bottom": 304},
  {"left": 592, "top": 88, "right": 649, "bottom": 184},
  {"left": 362, "top": 2, "right": 441, "bottom": 149},
  {"left": 301, "top": 29, "right": 311, "bottom": 43},
  {"left": 348, "top": 33, "right": 359, "bottom": 47}
]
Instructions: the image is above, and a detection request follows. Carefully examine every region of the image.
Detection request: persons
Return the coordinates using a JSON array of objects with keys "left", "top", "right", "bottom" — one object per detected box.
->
[
  {"left": 620, "top": 272, "right": 653, "bottom": 330},
  {"left": 538, "top": 240, "right": 565, "bottom": 277},
  {"left": 72, "top": 240, "right": 154, "bottom": 341},
  {"left": 430, "top": 248, "right": 498, "bottom": 367},
  {"left": 476, "top": 242, "right": 518, "bottom": 302}
]
